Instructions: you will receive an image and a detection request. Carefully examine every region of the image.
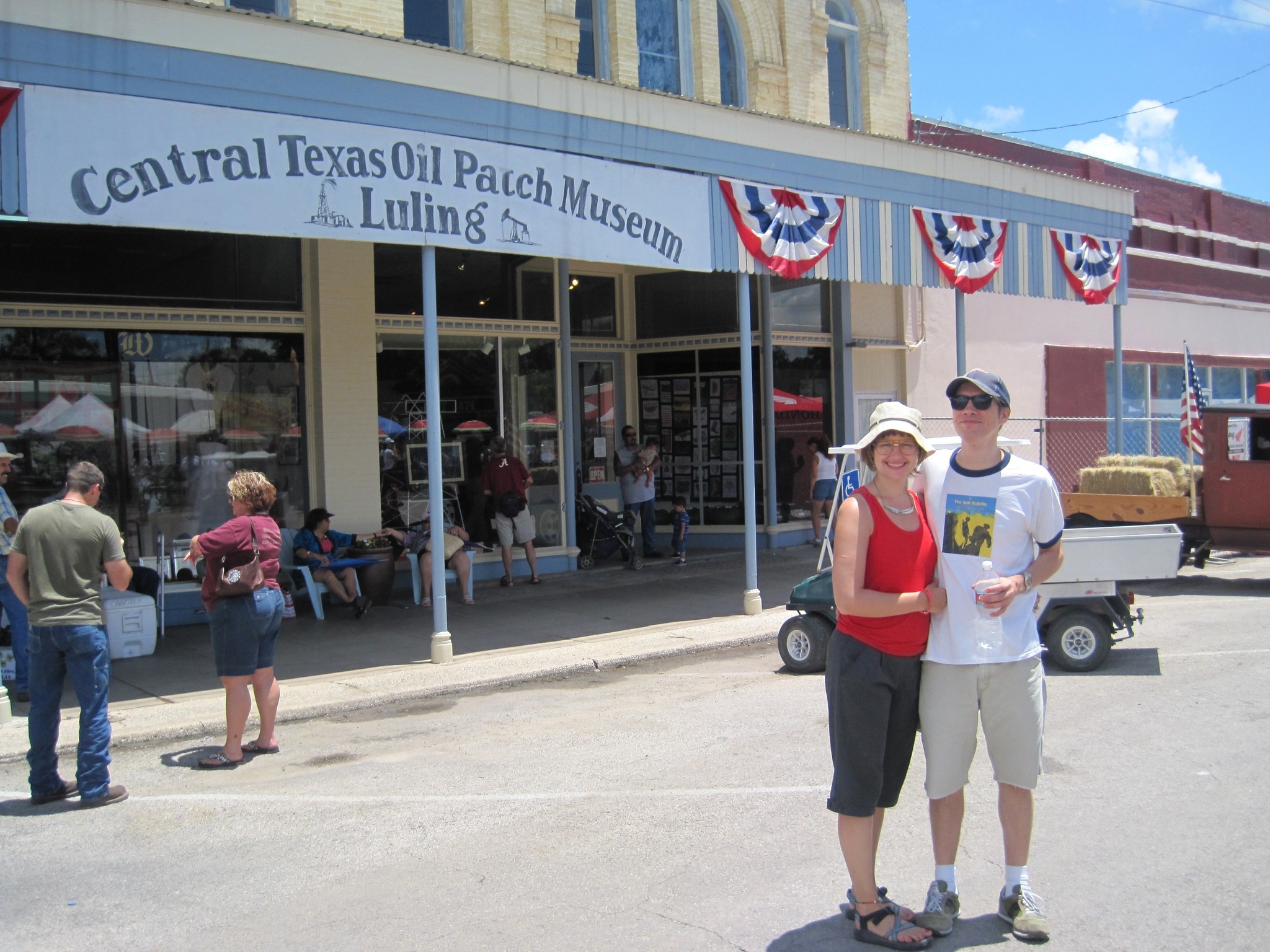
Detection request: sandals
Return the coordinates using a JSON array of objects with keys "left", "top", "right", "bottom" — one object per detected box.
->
[
  {"left": 846, "top": 887, "right": 918, "bottom": 922},
  {"left": 420, "top": 598, "right": 431, "bottom": 606},
  {"left": 531, "top": 577, "right": 541, "bottom": 584},
  {"left": 503, "top": 579, "right": 515, "bottom": 587},
  {"left": 853, "top": 905, "right": 934, "bottom": 951},
  {"left": 462, "top": 595, "right": 474, "bottom": 604}
]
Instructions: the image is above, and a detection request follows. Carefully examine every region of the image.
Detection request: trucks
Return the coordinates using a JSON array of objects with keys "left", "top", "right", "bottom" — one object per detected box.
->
[
  {"left": 1058, "top": 380, "right": 1270, "bottom": 576},
  {"left": 777, "top": 437, "right": 1185, "bottom": 673}
]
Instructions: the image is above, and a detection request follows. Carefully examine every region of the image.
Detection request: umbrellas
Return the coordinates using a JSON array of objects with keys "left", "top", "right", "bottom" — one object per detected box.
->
[
  {"left": 378, "top": 403, "right": 614, "bottom": 440},
  {"left": 0, "top": 393, "right": 301, "bottom": 460}
]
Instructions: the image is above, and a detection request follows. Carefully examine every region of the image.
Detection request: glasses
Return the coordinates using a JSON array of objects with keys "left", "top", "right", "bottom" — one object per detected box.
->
[
  {"left": 90, "top": 481, "right": 106, "bottom": 497},
  {"left": 949, "top": 393, "right": 996, "bottom": 410},
  {"left": 871, "top": 439, "right": 919, "bottom": 455},
  {"left": 324, "top": 518, "right": 329, "bottom": 522},
  {"left": 625, "top": 432, "right": 636, "bottom": 437}
]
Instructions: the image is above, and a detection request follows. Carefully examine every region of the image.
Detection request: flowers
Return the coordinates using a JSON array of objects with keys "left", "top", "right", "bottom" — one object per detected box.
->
[{"left": 356, "top": 533, "right": 392, "bottom": 550}]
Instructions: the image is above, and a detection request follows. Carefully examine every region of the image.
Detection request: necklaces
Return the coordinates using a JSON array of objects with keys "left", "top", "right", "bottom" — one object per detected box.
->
[{"left": 873, "top": 478, "right": 915, "bottom": 516}]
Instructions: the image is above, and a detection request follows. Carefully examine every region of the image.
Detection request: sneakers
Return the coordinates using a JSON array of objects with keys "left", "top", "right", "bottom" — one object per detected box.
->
[
  {"left": 917, "top": 880, "right": 962, "bottom": 936},
  {"left": 997, "top": 884, "right": 1052, "bottom": 941}
]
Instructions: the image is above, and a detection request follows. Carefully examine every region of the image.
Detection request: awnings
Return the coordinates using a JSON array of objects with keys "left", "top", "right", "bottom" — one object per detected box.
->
[{"left": 772, "top": 387, "right": 824, "bottom": 414}]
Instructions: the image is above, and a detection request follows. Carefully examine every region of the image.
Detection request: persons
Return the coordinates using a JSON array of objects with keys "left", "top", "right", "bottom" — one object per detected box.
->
[
  {"left": 671, "top": 496, "right": 690, "bottom": 566},
  {"left": 806, "top": 433, "right": 840, "bottom": 548},
  {"left": 183, "top": 472, "right": 285, "bottom": 770},
  {"left": 612, "top": 425, "right": 666, "bottom": 561},
  {"left": 5, "top": 461, "right": 133, "bottom": 807},
  {"left": 0, "top": 443, "right": 32, "bottom": 703},
  {"left": 383, "top": 510, "right": 474, "bottom": 606},
  {"left": 379, "top": 438, "right": 494, "bottom": 553},
  {"left": 293, "top": 508, "right": 389, "bottom": 620},
  {"left": 824, "top": 400, "right": 947, "bottom": 951},
  {"left": 916, "top": 366, "right": 1066, "bottom": 942},
  {"left": 483, "top": 435, "right": 540, "bottom": 588}
]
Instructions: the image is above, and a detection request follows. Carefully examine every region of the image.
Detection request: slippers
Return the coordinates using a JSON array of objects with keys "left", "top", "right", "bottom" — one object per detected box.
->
[
  {"left": 241, "top": 740, "right": 280, "bottom": 754},
  {"left": 198, "top": 753, "right": 247, "bottom": 769}
]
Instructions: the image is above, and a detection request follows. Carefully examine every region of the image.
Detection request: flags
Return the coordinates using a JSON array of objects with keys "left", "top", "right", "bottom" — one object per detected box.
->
[{"left": 1181, "top": 343, "right": 1205, "bottom": 455}]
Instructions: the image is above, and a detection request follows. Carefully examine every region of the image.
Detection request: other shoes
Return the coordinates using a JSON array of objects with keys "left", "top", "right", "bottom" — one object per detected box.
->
[
  {"left": 670, "top": 552, "right": 680, "bottom": 558},
  {"left": 674, "top": 559, "right": 687, "bottom": 566},
  {"left": 31, "top": 780, "right": 80, "bottom": 804},
  {"left": 16, "top": 692, "right": 30, "bottom": 702},
  {"left": 643, "top": 552, "right": 663, "bottom": 558},
  {"left": 806, "top": 539, "right": 822, "bottom": 547},
  {"left": 620, "top": 554, "right": 636, "bottom": 562},
  {"left": 345, "top": 596, "right": 373, "bottom": 621},
  {"left": 79, "top": 785, "right": 129, "bottom": 810}
]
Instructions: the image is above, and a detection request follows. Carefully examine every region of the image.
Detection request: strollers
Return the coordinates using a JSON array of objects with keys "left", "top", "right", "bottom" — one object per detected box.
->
[{"left": 576, "top": 491, "right": 644, "bottom": 570}]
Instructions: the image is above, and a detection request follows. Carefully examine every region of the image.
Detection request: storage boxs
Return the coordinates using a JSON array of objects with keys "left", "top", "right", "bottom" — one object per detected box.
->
[
  {"left": 154, "top": 581, "right": 211, "bottom": 628},
  {"left": 99, "top": 586, "right": 158, "bottom": 661},
  {"left": 0, "top": 646, "right": 17, "bottom": 680}
]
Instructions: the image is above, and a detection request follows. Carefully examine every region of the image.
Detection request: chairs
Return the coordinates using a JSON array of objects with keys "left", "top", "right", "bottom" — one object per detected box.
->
[
  {"left": 405, "top": 522, "right": 477, "bottom": 606},
  {"left": 277, "top": 528, "right": 368, "bottom": 620}
]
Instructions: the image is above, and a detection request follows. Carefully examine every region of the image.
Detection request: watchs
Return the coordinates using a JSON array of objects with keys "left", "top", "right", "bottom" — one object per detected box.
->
[{"left": 1018, "top": 571, "right": 1033, "bottom": 592}]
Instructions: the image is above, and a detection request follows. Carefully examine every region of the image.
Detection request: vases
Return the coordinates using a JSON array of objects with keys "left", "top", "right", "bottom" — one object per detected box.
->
[{"left": 345, "top": 544, "right": 395, "bottom": 606}]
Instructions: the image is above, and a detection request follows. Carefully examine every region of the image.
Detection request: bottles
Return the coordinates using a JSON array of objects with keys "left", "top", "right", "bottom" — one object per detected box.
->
[{"left": 972, "top": 560, "right": 1003, "bottom": 649}]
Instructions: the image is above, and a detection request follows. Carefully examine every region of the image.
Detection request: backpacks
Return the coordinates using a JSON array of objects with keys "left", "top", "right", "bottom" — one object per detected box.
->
[{"left": 379, "top": 456, "right": 385, "bottom": 467}]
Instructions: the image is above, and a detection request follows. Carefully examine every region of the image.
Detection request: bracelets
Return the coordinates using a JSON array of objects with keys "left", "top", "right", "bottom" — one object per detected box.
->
[
  {"left": 307, "top": 551, "right": 310, "bottom": 560},
  {"left": 632, "top": 465, "right": 633, "bottom": 469},
  {"left": 643, "top": 469, "right": 646, "bottom": 474},
  {"left": 921, "top": 590, "right": 933, "bottom": 615},
  {"left": 373, "top": 533, "right": 376, "bottom": 539},
  {"left": 646, "top": 467, "right": 649, "bottom": 472}
]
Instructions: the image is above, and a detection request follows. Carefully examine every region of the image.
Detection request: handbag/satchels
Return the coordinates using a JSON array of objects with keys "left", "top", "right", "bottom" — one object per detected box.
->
[
  {"left": 215, "top": 516, "right": 265, "bottom": 596},
  {"left": 499, "top": 492, "right": 526, "bottom": 517},
  {"left": 425, "top": 532, "right": 464, "bottom": 560},
  {"left": 278, "top": 583, "right": 296, "bottom": 620}
]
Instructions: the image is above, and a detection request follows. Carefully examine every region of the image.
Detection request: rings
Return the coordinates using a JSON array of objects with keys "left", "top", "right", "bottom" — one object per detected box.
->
[
  {"left": 643, "top": 460, "right": 645, "bottom": 461},
  {"left": 644, "top": 462, "right": 645, "bottom": 464},
  {"left": 636, "top": 474, "right": 637, "bottom": 476},
  {"left": 636, "top": 473, "right": 637, "bottom": 474}
]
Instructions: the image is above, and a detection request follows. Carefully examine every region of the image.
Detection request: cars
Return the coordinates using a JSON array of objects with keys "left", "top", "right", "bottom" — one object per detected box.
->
[{"left": 409, "top": 465, "right": 563, "bottom": 548}]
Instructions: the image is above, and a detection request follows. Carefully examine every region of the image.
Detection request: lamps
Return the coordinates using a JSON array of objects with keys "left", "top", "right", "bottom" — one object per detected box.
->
[
  {"left": 517, "top": 337, "right": 531, "bottom": 355},
  {"left": 375, "top": 332, "right": 384, "bottom": 353},
  {"left": 481, "top": 336, "right": 495, "bottom": 356}
]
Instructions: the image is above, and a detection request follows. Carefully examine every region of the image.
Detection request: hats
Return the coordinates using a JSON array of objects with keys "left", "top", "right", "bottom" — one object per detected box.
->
[
  {"left": 0, "top": 442, "right": 24, "bottom": 459},
  {"left": 385, "top": 438, "right": 395, "bottom": 444},
  {"left": 422, "top": 511, "right": 430, "bottom": 520},
  {"left": 946, "top": 368, "right": 1011, "bottom": 411},
  {"left": 853, "top": 401, "right": 936, "bottom": 459},
  {"left": 308, "top": 508, "right": 335, "bottom": 519}
]
